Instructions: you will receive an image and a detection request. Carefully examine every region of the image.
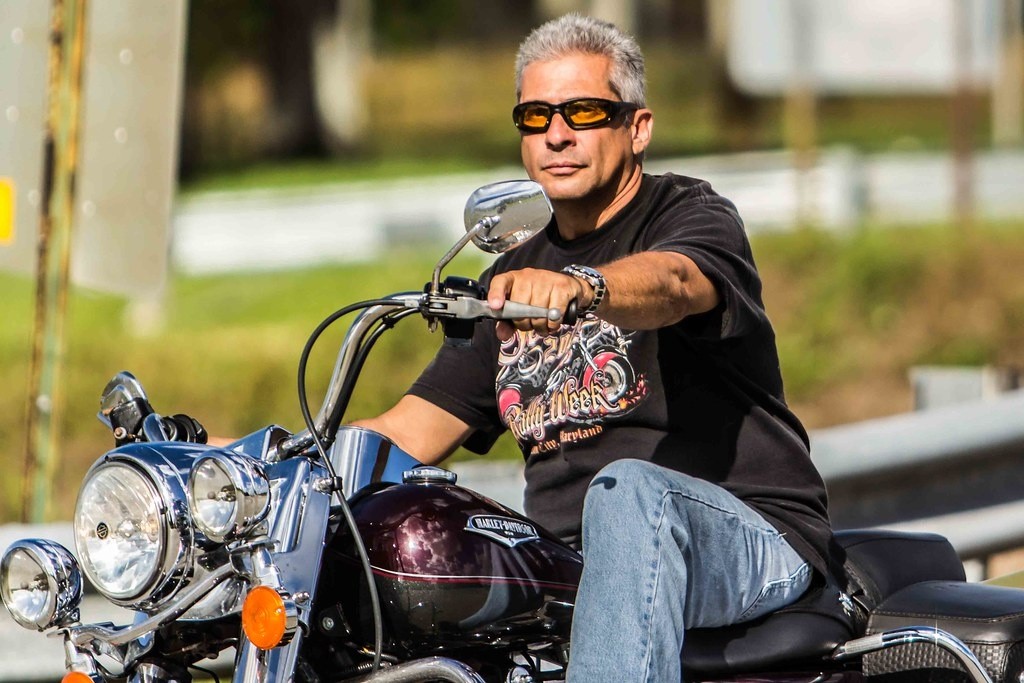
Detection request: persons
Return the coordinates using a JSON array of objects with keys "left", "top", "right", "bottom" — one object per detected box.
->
[{"left": 205, "top": 15, "right": 835, "bottom": 683}]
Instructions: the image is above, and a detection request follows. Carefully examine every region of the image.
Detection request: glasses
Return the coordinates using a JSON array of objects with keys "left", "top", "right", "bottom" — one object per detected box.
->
[{"left": 512, "top": 98, "right": 638, "bottom": 133}]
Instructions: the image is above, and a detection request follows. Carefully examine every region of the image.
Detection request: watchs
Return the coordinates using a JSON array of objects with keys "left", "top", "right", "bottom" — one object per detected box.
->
[{"left": 560, "top": 263, "right": 607, "bottom": 318}]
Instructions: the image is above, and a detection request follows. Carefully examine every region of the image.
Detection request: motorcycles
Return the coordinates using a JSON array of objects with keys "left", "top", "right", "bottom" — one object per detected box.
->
[{"left": 0, "top": 182, "right": 1024, "bottom": 683}]
